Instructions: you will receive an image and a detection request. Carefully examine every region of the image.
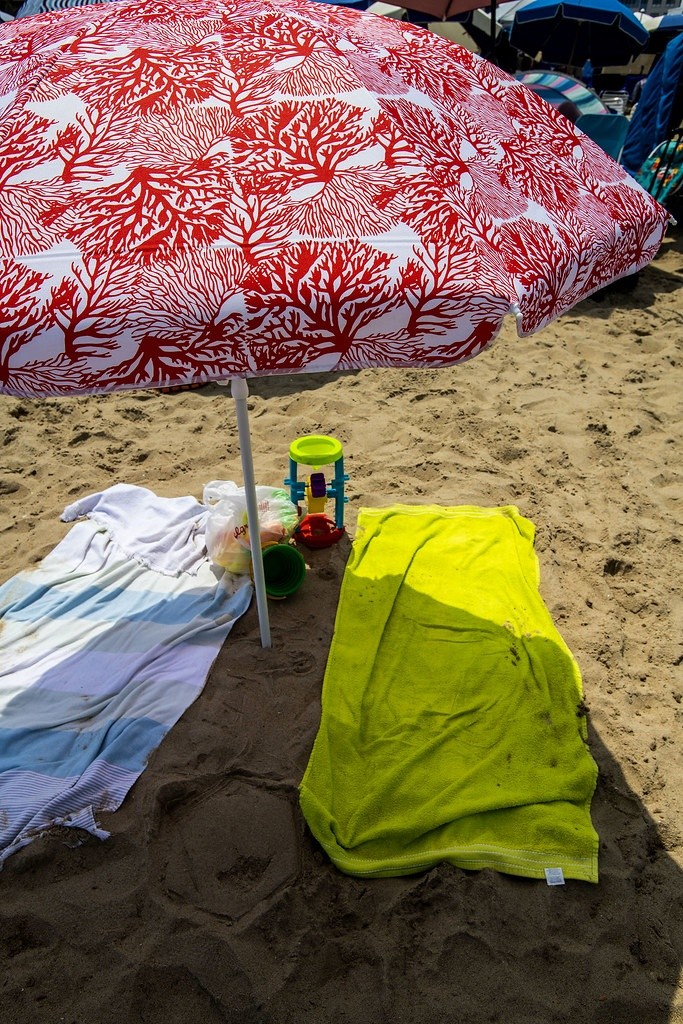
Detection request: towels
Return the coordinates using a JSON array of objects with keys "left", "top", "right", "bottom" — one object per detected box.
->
[
  {"left": 296, "top": 500, "right": 606, "bottom": 885},
  {"left": 0, "top": 480, "right": 254, "bottom": 865}
]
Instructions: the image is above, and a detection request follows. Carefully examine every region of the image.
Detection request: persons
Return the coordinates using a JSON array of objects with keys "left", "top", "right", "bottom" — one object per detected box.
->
[{"left": 16, "top": 0, "right": 518, "bottom": 100}]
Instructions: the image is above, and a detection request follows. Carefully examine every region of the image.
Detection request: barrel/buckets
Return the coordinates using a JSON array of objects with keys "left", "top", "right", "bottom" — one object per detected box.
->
[{"left": 249, "top": 541, "right": 306, "bottom": 600}]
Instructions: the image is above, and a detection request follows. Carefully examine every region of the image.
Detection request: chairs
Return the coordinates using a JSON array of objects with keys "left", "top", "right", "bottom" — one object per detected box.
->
[{"left": 574, "top": 113, "right": 630, "bottom": 165}]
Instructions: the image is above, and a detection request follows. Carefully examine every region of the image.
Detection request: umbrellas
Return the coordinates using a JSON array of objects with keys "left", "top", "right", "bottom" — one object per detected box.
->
[
  {"left": 0, "top": 0, "right": 675, "bottom": 647},
  {"left": 509, "top": 0, "right": 651, "bottom": 66},
  {"left": 647, "top": 14, "right": 683, "bottom": 53}
]
